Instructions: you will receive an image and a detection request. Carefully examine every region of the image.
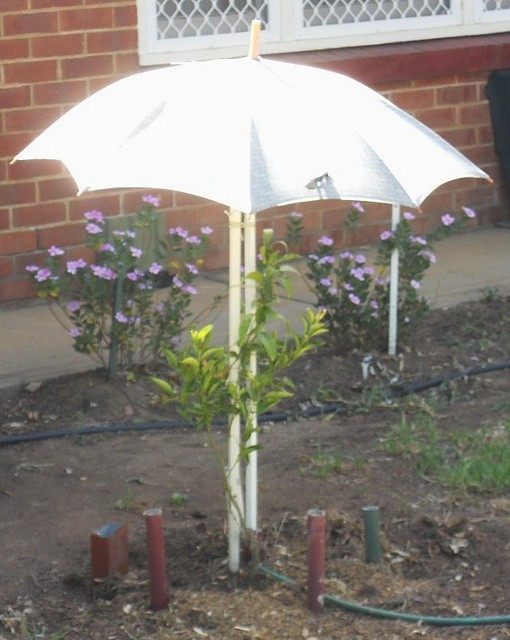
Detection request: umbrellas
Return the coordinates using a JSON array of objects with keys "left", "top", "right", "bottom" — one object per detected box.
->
[{"left": 10, "top": 20, "right": 494, "bottom": 573}]
